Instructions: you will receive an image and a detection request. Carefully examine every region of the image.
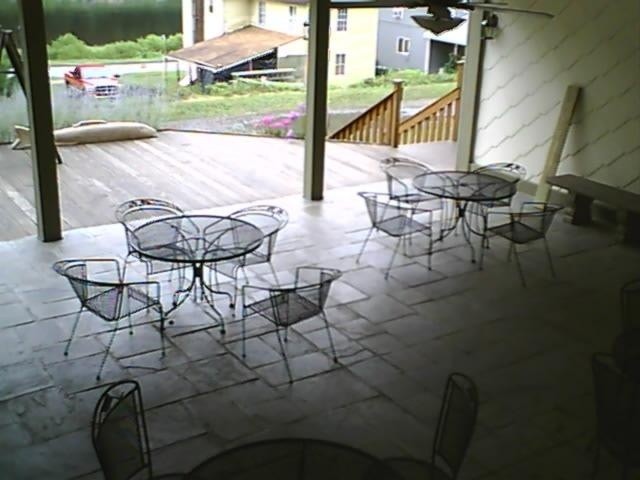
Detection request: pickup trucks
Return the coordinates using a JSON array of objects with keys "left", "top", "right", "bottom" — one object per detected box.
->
[{"left": 63, "top": 65, "right": 124, "bottom": 101}]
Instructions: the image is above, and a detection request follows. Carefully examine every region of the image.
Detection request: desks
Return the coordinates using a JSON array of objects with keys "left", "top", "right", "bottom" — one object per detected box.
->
[
  {"left": 181, "top": 438, "right": 404, "bottom": 478},
  {"left": 612, "top": 331, "right": 639, "bottom": 378}
]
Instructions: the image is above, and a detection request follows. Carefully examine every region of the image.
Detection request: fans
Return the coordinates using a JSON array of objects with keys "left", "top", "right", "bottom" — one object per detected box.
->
[{"left": 319, "top": 0, "right": 555, "bottom": 36}]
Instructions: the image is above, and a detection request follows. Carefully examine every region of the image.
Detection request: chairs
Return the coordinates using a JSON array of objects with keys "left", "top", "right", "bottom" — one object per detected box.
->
[
  {"left": 88, "top": 379, "right": 186, "bottom": 480},
  {"left": 356, "top": 152, "right": 564, "bottom": 289},
  {"left": 387, "top": 373, "right": 487, "bottom": 480},
  {"left": 586, "top": 350, "right": 639, "bottom": 480},
  {"left": 619, "top": 276, "right": 640, "bottom": 336}
]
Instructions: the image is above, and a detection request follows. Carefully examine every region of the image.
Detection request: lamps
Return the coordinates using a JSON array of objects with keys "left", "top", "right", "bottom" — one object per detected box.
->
[{"left": 480, "top": 14, "right": 498, "bottom": 40}]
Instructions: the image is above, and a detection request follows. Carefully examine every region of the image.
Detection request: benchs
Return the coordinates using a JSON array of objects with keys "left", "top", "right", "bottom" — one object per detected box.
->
[{"left": 544, "top": 173, "right": 640, "bottom": 240}]
